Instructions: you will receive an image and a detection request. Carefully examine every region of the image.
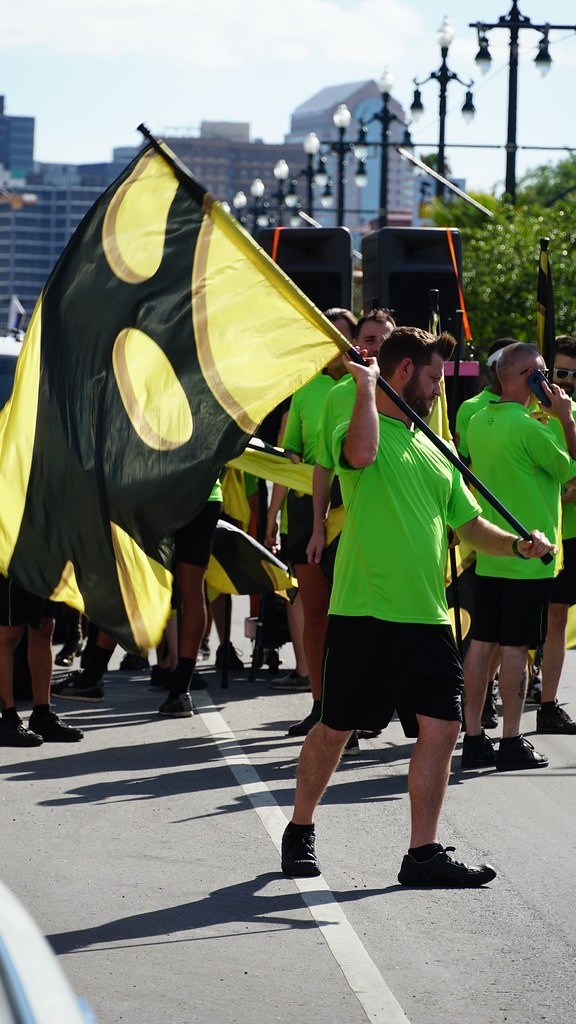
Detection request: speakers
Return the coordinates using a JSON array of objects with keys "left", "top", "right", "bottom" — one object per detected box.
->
[
  {"left": 360, "top": 227, "right": 466, "bottom": 360},
  {"left": 255, "top": 226, "right": 353, "bottom": 320}
]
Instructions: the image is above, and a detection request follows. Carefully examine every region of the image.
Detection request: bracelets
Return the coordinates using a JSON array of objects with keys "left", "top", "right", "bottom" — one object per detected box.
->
[{"left": 511, "top": 537, "right": 531, "bottom": 560}]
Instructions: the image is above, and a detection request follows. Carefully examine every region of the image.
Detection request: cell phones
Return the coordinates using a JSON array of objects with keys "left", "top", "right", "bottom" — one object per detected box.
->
[{"left": 527, "top": 370, "right": 555, "bottom": 410}]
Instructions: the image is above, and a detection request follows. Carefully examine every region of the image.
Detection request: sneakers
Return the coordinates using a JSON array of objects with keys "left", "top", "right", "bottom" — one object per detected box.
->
[
  {"left": 398, "top": 847, "right": 496, "bottom": 886},
  {"left": 460, "top": 732, "right": 497, "bottom": 768},
  {"left": 281, "top": 830, "right": 320, "bottom": 876},
  {"left": 536, "top": 699, "right": 576, "bottom": 734},
  {"left": 496, "top": 734, "right": 549, "bottom": 771}
]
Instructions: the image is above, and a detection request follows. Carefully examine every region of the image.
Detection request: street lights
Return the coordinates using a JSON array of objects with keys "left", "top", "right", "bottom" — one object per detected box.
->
[
  {"left": 410, "top": 12, "right": 476, "bottom": 200},
  {"left": 353, "top": 70, "right": 416, "bottom": 229},
  {"left": 221, "top": 104, "right": 368, "bottom": 238},
  {"left": 469, "top": 0, "right": 576, "bottom": 205}
]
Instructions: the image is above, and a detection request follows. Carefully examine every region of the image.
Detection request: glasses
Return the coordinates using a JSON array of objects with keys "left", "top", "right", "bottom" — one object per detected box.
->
[
  {"left": 520, "top": 368, "right": 550, "bottom": 378},
  {"left": 553, "top": 368, "right": 576, "bottom": 379}
]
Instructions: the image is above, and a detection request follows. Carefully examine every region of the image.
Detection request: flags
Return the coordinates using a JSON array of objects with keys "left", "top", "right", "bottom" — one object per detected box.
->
[{"left": 0, "top": 140, "right": 358, "bottom": 652}]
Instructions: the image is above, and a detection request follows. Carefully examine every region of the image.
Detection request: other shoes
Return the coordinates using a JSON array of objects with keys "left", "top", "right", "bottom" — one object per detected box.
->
[
  {"left": 479, "top": 695, "right": 499, "bottom": 729},
  {"left": 344, "top": 730, "right": 360, "bottom": 756},
  {"left": 157, "top": 692, "right": 195, "bottom": 717},
  {"left": 288, "top": 713, "right": 321, "bottom": 735},
  {"left": 53, "top": 639, "right": 313, "bottom": 700},
  {"left": 27, "top": 713, "right": 83, "bottom": 741},
  {"left": 0, "top": 719, "right": 43, "bottom": 746}
]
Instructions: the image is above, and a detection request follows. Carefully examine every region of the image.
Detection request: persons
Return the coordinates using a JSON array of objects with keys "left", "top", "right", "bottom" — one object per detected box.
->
[
  {"left": 0, "top": 472, "right": 377, "bottom": 748},
  {"left": 263, "top": 305, "right": 359, "bottom": 692},
  {"left": 456, "top": 335, "right": 576, "bottom": 771},
  {"left": 287, "top": 307, "right": 398, "bottom": 757},
  {"left": 282, "top": 327, "right": 558, "bottom": 888}
]
